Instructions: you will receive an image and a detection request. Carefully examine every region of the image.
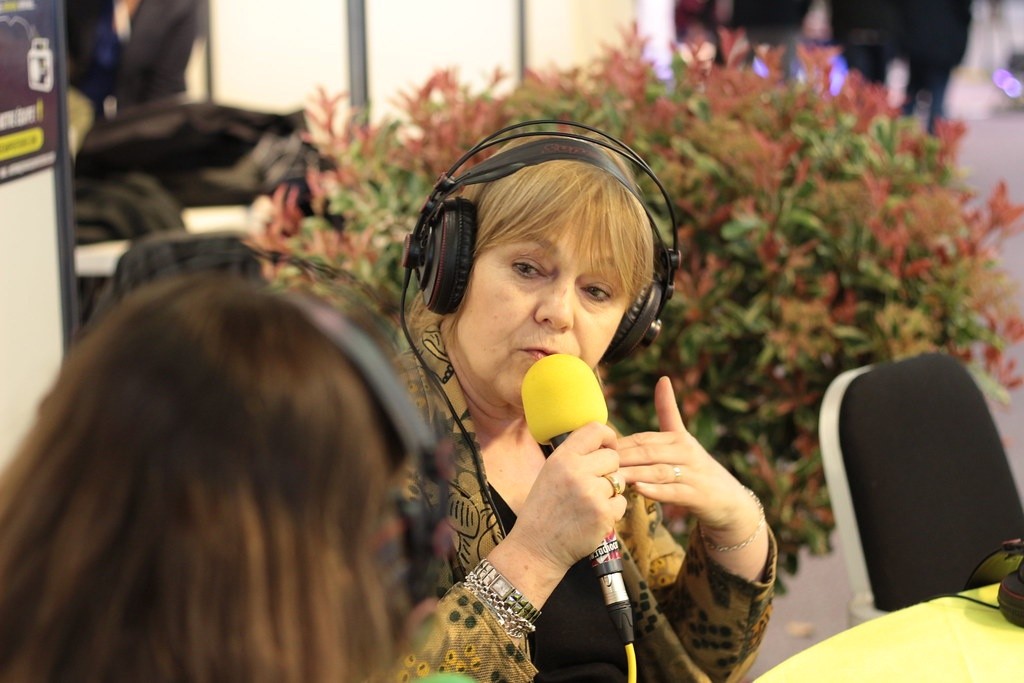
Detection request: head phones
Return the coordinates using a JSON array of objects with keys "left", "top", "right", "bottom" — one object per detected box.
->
[
  {"left": 89, "top": 234, "right": 455, "bottom": 617},
  {"left": 962, "top": 537, "right": 1024, "bottom": 627},
  {"left": 401, "top": 119, "right": 680, "bottom": 367}
]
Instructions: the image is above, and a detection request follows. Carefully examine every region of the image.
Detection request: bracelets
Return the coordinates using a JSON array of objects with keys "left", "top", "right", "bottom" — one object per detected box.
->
[
  {"left": 460, "top": 557, "right": 542, "bottom": 640},
  {"left": 696, "top": 484, "right": 766, "bottom": 552}
]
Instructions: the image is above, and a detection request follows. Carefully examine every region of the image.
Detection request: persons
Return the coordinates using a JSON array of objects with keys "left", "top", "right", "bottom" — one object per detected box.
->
[
  {"left": 387, "top": 119, "right": 779, "bottom": 683},
  {"left": 701, "top": 0, "right": 973, "bottom": 133},
  {"left": 64, "top": 0, "right": 200, "bottom": 118},
  {"left": 0, "top": 269, "right": 420, "bottom": 683}
]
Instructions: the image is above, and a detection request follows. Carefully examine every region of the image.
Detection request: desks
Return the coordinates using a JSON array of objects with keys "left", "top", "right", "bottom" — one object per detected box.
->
[{"left": 751, "top": 582, "right": 1023, "bottom": 682}]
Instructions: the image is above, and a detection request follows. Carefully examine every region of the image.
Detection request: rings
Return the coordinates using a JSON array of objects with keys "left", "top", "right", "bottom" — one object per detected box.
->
[
  {"left": 604, "top": 474, "right": 621, "bottom": 497},
  {"left": 672, "top": 465, "right": 681, "bottom": 483}
]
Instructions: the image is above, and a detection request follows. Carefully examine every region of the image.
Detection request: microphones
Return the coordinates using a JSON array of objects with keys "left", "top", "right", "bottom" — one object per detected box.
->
[{"left": 522, "top": 353, "right": 634, "bottom": 646}]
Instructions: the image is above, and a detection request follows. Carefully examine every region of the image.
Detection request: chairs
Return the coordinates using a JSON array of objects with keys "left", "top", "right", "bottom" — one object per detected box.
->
[{"left": 818, "top": 351, "right": 1023, "bottom": 626}]
